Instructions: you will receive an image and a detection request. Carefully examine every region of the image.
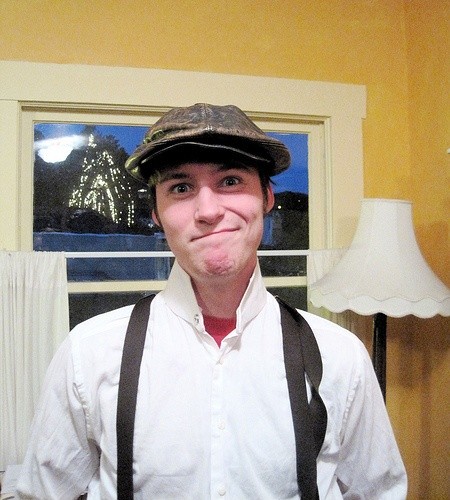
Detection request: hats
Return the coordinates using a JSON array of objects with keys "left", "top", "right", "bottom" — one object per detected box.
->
[{"left": 121, "top": 104, "right": 292, "bottom": 178}]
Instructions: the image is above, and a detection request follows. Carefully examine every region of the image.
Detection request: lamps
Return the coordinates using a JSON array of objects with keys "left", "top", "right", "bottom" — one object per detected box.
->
[{"left": 306, "top": 198, "right": 450, "bottom": 406}]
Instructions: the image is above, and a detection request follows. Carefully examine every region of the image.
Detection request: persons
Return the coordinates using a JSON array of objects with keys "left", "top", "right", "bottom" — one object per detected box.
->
[{"left": 13, "top": 103, "right": 410, "bottom": 500}]
